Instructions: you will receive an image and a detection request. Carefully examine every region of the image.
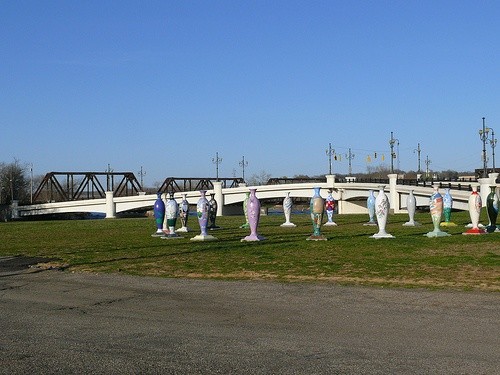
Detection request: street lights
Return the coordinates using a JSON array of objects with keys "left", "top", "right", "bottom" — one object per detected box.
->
[
  {"left": 212, "top": 152, "right": 223, "bottom": 182},
  {"left": 388, "top": 132, "right": 399, "bottom": 174},
  {"left": 137, "top": 166, "right": 147, "bottom": 192},
  {"left": 239, "top": 155, "right": 248, "bottom": 178},
  {"left": 325, "top": 143, "right": 335, "bottom": 174},
  {"left": 479, "top": 117, "right": 497, "bottom": 178},
  {"left": 344, "top": 148, "right": 355, "bottom": 177},
  {"left": 413, "top": 142, "right": 431, "bottom": 178}
]
